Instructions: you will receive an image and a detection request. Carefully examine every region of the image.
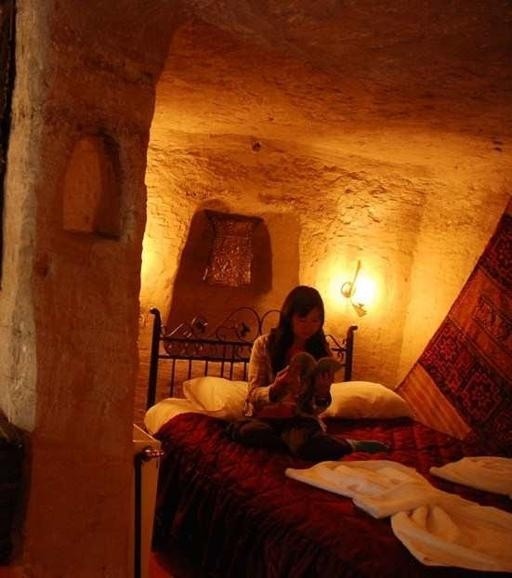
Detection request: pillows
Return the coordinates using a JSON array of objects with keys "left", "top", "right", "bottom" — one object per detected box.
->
[{"left": 182, "top": 375, "right": 416, "bottom": 422}]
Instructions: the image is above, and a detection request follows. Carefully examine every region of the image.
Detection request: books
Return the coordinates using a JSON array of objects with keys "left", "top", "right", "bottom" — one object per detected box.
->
[{"left": 287, "top": 353, "right": 344, "bottom": 386}]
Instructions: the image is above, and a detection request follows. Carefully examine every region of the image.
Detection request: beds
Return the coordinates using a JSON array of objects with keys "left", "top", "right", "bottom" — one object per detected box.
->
[{"left": 148, "top": 306, "right": 512, "bottom": 577}]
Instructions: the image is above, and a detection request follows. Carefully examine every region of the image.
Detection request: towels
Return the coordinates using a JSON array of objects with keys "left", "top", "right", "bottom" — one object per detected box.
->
[{"left": 284, "top": 456, "right": 511, "bottom": 573}]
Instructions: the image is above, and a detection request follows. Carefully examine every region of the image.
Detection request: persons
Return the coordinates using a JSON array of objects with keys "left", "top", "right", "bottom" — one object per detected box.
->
[{"left": 220, "top": 283, "right": 393, "bottom": 461}]
